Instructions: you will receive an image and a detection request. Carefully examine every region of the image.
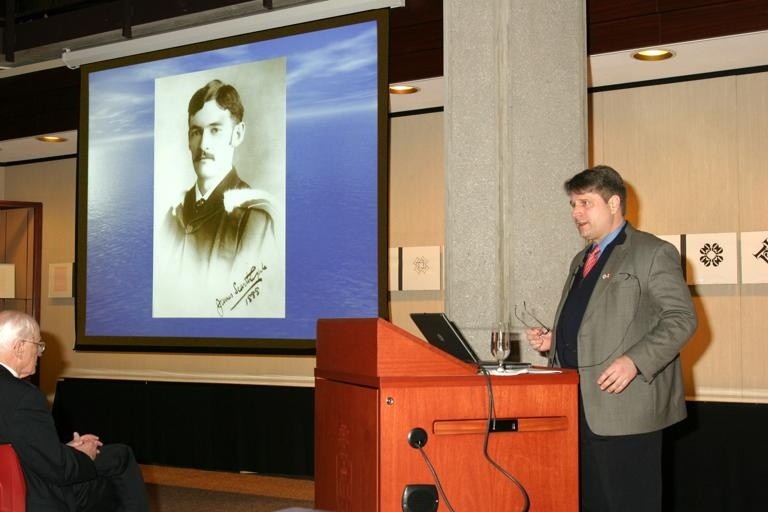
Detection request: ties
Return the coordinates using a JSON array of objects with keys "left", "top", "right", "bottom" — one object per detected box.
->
[{"left": 582, "top": 245, "right": 600, "bottom": 278}]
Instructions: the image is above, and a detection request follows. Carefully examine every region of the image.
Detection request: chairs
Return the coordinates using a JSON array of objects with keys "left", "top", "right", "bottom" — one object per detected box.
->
[{"left": 0, "top": 442, "right": 29, "bottom": 511}]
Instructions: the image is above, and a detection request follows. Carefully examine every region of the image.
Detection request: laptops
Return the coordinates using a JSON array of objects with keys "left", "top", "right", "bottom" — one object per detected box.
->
[{"left": 409, "top": 312, "right": 533, "bottom": 370}]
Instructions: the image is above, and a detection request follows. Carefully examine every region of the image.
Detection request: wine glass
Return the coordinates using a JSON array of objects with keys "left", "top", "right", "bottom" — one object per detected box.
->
[{"left": 490, "top": 321, "right": 511, "bottom": 374}]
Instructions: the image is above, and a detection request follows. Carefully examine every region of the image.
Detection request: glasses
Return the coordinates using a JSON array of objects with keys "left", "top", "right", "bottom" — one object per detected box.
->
[{"left": 13, "top": 340, "right": 46, "bottom": 353}]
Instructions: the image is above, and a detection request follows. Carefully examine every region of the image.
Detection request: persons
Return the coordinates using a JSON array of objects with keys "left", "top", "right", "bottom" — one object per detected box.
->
[
  {"left": 520, "top": 164, "right": 699, "bottom": 511},
  {"left": 1, "top": 307, "right": 158, "bottom": 512},
  {"left": 162, "top": 77, "right": 281, "bottom": 278}
]
